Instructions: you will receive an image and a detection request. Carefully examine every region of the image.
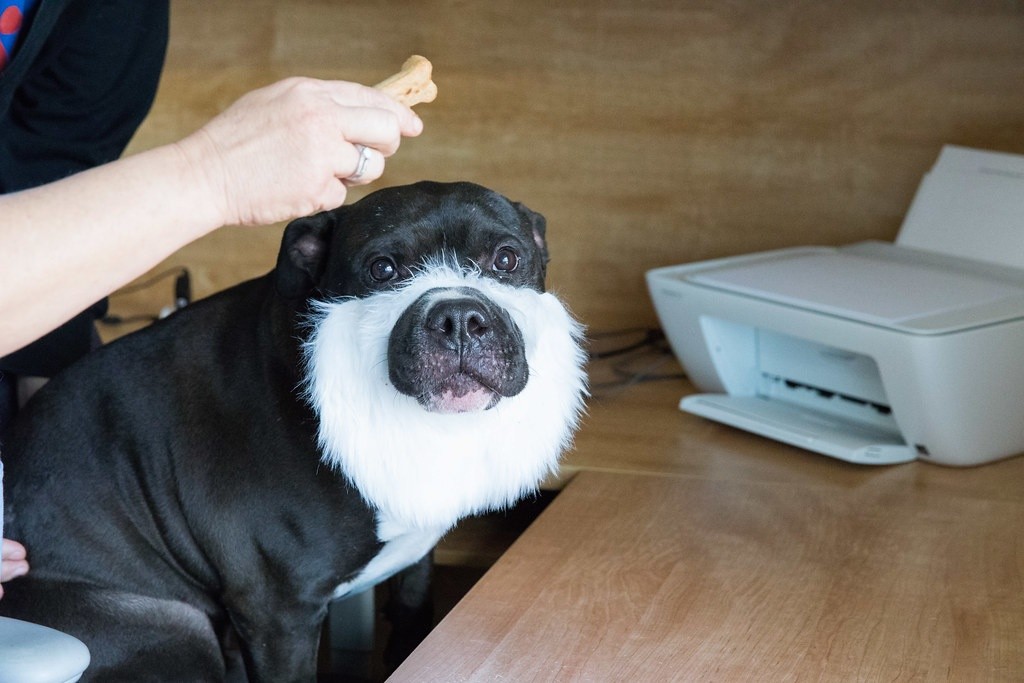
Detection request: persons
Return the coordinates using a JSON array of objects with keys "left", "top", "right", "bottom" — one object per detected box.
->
[{"left": 0, "top": 0, "right": 441, "bottom": 610}]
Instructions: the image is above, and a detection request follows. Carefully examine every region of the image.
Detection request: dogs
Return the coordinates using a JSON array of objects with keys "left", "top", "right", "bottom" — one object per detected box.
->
[{"left": 0, "top": 179, "right": 590, "bottom": 683}]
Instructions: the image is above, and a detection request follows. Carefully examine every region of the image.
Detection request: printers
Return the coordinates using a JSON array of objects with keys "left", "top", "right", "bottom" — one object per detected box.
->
[{"left": 644, "top": 144, "right": 1024, "bottom": 467}]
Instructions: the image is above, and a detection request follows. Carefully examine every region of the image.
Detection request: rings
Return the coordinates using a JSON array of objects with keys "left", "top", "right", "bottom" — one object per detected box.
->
[{"left": 344, "top": 143, "right": 372, "bottom": 180}]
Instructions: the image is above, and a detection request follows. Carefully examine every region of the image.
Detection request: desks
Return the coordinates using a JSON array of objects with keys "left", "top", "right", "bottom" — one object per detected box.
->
[{"left": 381, "top": 355, "right": 1024, "bottom": 683}]
